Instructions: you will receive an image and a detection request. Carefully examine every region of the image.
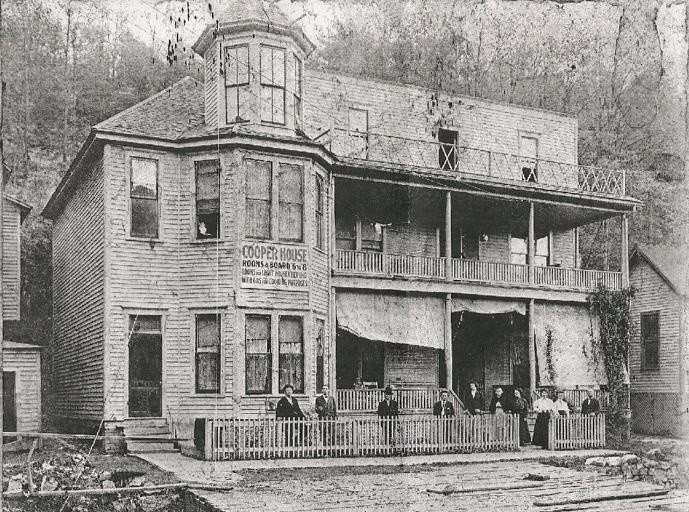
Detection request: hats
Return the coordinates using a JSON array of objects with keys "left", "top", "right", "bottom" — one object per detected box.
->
[{"left": 382, "top": 387, "right": 393, "bottom": 394}]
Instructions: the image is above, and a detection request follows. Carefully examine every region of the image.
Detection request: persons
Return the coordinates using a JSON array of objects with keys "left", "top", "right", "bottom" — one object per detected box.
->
[
  {"left": 378, "top": 387, "right": 400, "bottom": 447},
  {"left": 315, "top": 382, "right": 337, "bottom": 446},
  {"left": 490, "top": 386, "right": 601, "bottom": 450},
  {"left": 464, "top": 380, "right": 484, "bottom": 417},
  {"left": 276, "top": 383, "right": 312, "bottom": 446},
  {"left": 430, "top": 389, "right": 457, "bottom": 443}
]
